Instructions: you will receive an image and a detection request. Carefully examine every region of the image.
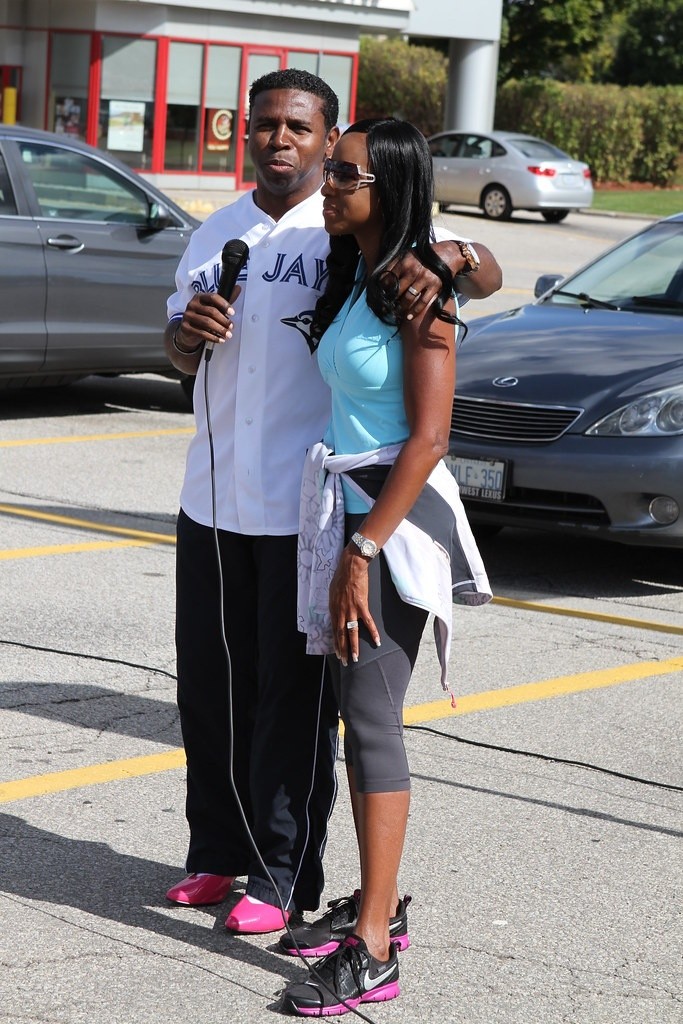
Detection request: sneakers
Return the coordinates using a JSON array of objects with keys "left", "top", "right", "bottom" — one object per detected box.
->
[
  {"left": 282, "top": 935, "right": 401, "bottom": 1016},
  {"left": 279, "top": 887, "right": 413, "bottom": 957}
]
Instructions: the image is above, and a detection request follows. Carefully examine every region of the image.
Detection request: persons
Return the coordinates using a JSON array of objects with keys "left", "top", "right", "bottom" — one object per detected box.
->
[
  {"left": 164, "top": 68, "right": 503, "bottom": 934},
  {"left": 279, "top": 117, "right": 459, "bottom": 1017}
]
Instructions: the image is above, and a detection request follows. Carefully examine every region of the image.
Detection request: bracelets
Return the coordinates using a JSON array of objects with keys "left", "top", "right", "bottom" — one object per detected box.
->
[{"left": 173, "top": 322, "right": 205, "bottom": 355}]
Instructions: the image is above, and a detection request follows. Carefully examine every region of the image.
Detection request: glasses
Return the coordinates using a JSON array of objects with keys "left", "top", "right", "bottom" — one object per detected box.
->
[{"left": 322, "top": 158, "right": 379, "bottom": 192}]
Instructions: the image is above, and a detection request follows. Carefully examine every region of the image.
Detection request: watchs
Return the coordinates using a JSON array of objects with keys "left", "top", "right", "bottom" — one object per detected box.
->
[
  {"left": 449, "top": 240, "right": 480, "bottom": 278},
  {"left": 351, "top": 531, "right": 380, "bottom": 560}
]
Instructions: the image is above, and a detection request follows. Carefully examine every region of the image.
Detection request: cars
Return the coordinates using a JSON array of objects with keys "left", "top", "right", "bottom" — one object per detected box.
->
[
  {"left": 425, "top": 131, "right": 594, "bottom": 223},
  {"left": 442, "top": 210, "right": 683, "bottom": 548},
  {"left": 0, "top": 124, "right": 203, "bottom": 398}
]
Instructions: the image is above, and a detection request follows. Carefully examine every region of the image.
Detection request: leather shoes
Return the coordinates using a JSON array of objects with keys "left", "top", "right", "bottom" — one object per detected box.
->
[
  {"left": 227, "top": 895, "right": 293, "bottom": 934},
  {"left": 165, "top": 872, "right": 235, "bottom": 906}
]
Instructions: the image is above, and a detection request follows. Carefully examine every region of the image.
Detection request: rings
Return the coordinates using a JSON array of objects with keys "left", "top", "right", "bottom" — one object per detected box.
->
[
  {"left": 407, "top": 287, "right": 420, "bottom": 296},
  {"left": 347, "top": 621, "right": 358, "bottom": 629}
]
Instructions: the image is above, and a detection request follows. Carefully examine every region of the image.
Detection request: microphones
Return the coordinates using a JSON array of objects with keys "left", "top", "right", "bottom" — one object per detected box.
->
[{"left": 203, "top": 240, "right": 249, "bottom": 363}]
